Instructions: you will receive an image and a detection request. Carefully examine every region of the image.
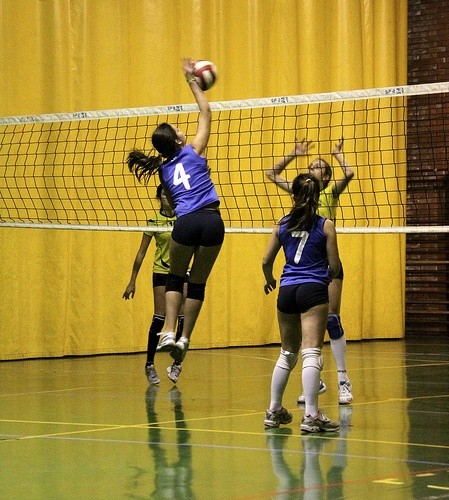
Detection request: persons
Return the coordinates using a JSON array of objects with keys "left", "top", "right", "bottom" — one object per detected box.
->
[
  {"left": 266, "top": 137, "right": 354, "bottom": 402},
  {"left": 261, "top": 174, "right": 340, "bottom": 433},
  {"left": 122, "top": 183, "right": 191, "bottom": 384},
  {"left": 269, "top": 434, "right": 347, "bottom": 500},
  {"left": 144, "top": 384, "right": 192, "bottom": 500},
  {"left": 126, "top": 56, "right": 225, "bottom": 360}
]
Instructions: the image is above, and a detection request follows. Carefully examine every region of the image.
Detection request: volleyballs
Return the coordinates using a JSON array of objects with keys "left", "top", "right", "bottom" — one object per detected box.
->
[{"left": 193, "top": 60, "right": 217, "bottom": 91}]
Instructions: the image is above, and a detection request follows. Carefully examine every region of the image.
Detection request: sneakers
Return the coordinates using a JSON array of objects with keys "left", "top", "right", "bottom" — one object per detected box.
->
[
  {"left": 300, "top": 411, "right": 340, "bottom": 432},
  {"left": 338, "top": 380, "right": 353, "bottom": 404},
  {"left": 156, "top": 331, "right": 175, "bottom": 352},
  {"left": 144, "top": 363, "right": 160, "bottom": 384},
  {"left": 297, "top": 380, "right": 327, "bottom": 402},
  {"left": 264, "top": 407, "right": 292, "bottom": 427},
  {"left": 170, "top": 337, "right": 189, "bottom": 363},
  {"left": 167, "top": 363, "right": 182, "bottom": 384}
]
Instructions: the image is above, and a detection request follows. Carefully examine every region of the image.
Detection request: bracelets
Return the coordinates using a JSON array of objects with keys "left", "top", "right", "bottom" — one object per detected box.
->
[{"left": 187, "top": 77, "right": 196, "bottom": 84}]
